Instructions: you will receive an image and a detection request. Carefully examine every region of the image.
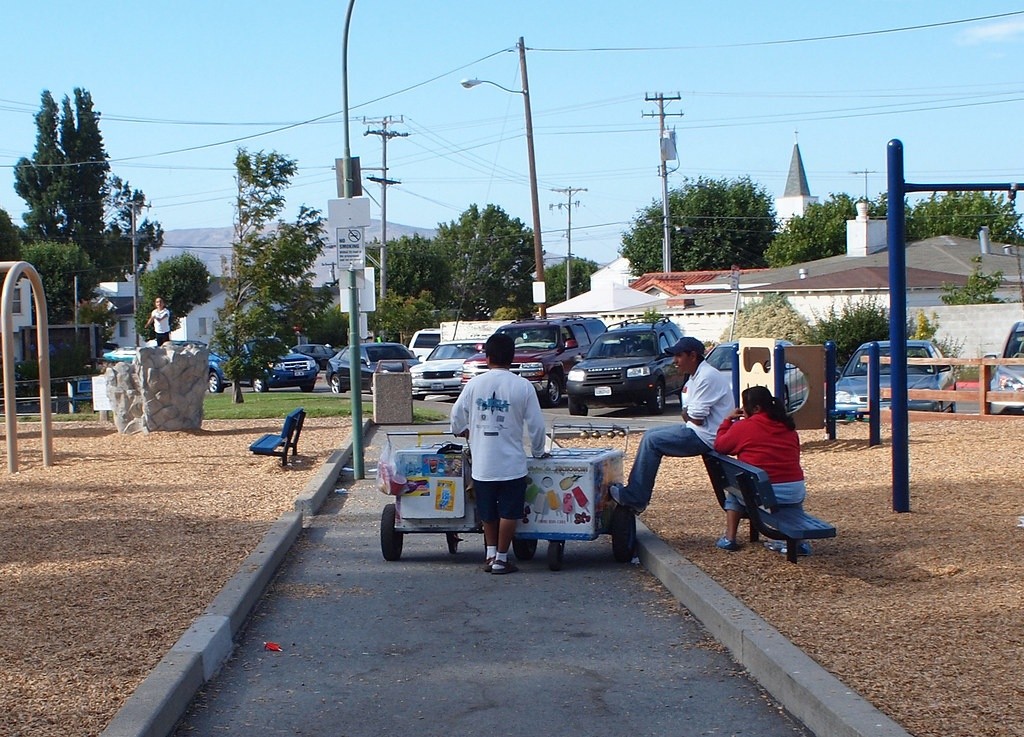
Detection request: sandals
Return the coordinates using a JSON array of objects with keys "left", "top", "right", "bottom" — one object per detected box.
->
[
  {"left": 485, "top": 556, "right": 496, "bottom": 572},
  {"left": 492, "top": 558, "right": 518, "bottom": 574}
]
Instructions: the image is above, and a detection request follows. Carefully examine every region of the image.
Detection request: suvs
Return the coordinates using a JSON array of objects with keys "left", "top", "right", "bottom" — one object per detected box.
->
[
  {"left": 460, "top": 314, "right": 609, "bottom": 409},
  {"left": 567, "top": 316, "right": 693, "bottom": 418}
]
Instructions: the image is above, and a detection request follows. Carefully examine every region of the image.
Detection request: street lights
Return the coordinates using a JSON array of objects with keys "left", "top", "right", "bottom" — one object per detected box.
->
[{"left": 462, "top": 78, "right": 548, "bottom": 320}]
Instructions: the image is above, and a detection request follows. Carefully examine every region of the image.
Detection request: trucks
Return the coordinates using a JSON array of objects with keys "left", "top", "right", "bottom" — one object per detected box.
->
[{"left": 409, "top": 319, "right": 519, "bottom": 363}]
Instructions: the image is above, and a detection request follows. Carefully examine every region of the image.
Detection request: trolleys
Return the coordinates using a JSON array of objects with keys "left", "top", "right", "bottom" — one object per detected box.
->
[
  {"left": 380, "top": 432, "right": 490, "bottom": 561},
  {"left": 511, "top": 425, "right": 636, "bottom": 571}
]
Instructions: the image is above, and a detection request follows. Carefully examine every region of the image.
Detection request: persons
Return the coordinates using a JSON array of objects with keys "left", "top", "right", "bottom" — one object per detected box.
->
[
  {"left": 145, "top": 297, "right": 171, "bottom": 346},
  {"left": 609, "top": 337, "right": 735, "bottom": 516},
  {"left": 450, "top": 333, "right": 551, "bottom": 574},
  {"left": 714, "top": 386, "right": 811, "bottom": 553}
]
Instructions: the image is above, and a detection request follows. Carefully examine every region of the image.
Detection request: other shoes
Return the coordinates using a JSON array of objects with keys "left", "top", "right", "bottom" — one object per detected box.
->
[{"left": 609, "top": 485, "right": 648, "bottom": 513}]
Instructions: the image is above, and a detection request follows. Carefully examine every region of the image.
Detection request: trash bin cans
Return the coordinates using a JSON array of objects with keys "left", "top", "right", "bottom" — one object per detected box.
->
[{"left": 371, "top": 359, "right": 414, "bottom": 424}]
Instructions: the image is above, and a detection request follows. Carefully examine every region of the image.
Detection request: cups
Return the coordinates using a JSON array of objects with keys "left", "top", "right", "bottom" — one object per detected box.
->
[{"left": 390, "top": 474, "right": 406, "bottom": 494}]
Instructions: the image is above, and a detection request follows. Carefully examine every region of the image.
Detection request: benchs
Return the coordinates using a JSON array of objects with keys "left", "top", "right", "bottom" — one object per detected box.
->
[
  {"left": 702, "top": 450, "right": 837, "bottom": 564},
  {"left": 249, "top": 408, "right": 307, "bottom": 466}
]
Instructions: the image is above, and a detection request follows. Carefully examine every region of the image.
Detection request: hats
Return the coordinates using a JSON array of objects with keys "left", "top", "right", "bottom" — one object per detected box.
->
[{"left": 664, "top": 336, "right": 706, "bottom": 356}]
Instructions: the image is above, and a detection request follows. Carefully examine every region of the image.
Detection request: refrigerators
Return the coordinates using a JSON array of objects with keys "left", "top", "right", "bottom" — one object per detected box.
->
[
  {"left": 395, "top": 443, "right": 480, "bottom": 528},
  {"left": 512, "top": 448, "right": 623, "bottom": 539}
]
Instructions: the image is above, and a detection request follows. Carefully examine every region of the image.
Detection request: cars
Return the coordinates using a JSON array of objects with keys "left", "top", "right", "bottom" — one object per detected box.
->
[
  {"left": 324, "top": 344, "right": 422, "bottom": 394},
  {"left": 409, "top": 339, "right": 488, "bottom": 402},
  {"left": 680, "top": 340, "right": 807, "bottom": 413},
  {"left": 824, "top": 340, "right": 958, "bottom": 418},
  {"left": 205, "top": 335, "right": 317, "bottom": 393},
  {"left": 291, "top": 344, "right": 337, "bottom": 371},
  {"left": 101, "top": 339, "right": 207, "bottom": 363},
  {"left": 983, "top": 321, "right": 1024, "bottom": 417}
]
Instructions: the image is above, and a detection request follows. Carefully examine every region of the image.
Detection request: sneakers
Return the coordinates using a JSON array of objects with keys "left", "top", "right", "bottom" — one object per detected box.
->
[
  {"left": 797, "top": 544, "right": 811, "bottom": 556},
  {"left": 715, "top": 537, "right": 736, "bottom": 553}
]
